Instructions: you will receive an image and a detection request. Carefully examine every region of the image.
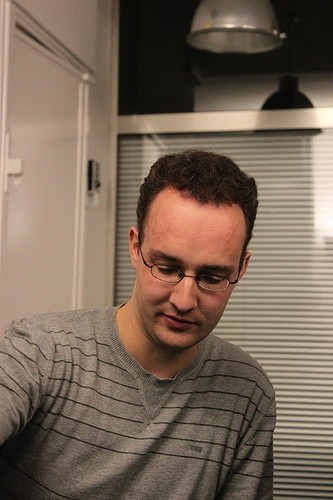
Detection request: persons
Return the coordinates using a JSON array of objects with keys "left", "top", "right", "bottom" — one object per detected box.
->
[{"left": 0, "top": 149, "right": 276, "bottom": 500}]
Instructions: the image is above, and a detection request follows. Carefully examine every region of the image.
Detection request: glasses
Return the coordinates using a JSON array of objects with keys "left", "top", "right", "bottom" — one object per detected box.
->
[{"left": 138, "top": 239, "right": 243, "bottom": 291}]
